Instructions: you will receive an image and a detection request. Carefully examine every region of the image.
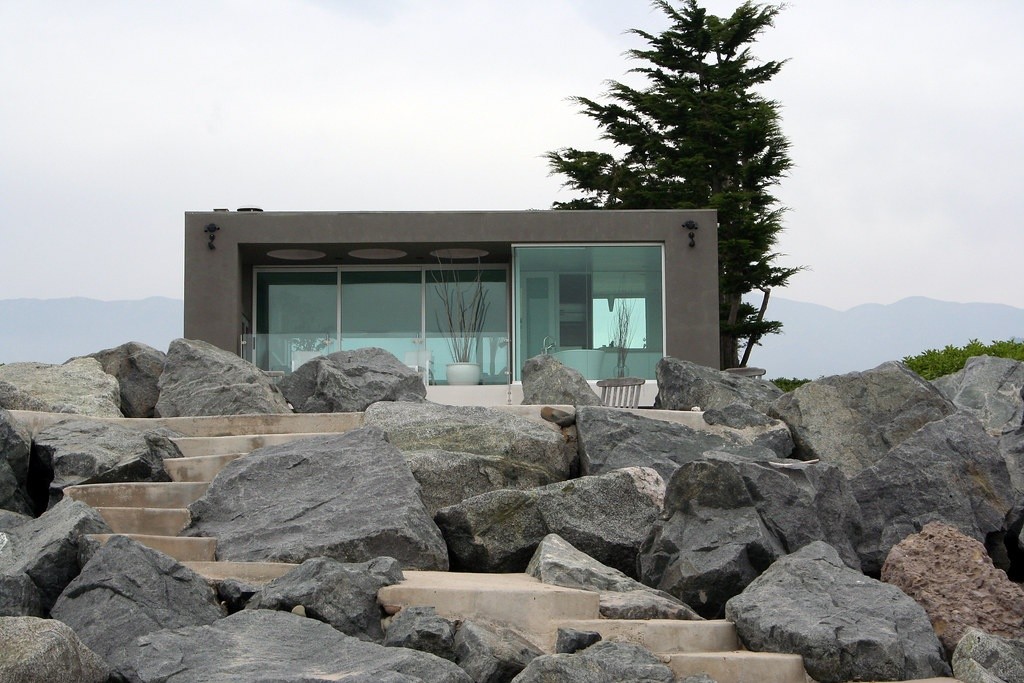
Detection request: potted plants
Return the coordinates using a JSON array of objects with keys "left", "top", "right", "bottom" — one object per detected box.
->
[{"left": 430, "top": 249, "right": 492, "bottom": 385}]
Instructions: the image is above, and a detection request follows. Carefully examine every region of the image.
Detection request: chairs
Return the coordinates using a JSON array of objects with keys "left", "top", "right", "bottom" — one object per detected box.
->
[{"left": 595, "top": 376, "right": 646, "bottom": 408}]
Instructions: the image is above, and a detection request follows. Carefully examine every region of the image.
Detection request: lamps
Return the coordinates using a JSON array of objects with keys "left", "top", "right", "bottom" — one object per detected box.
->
[
  {"left": 681, "top": 219, "right": 700, "bottom": 249},
  {"left": 203, "top": 222, "right": 222, "bottom": 253}
]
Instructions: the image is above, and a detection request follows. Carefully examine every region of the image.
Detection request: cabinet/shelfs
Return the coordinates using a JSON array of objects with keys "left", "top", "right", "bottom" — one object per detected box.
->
[{"left": 524, "top": 270, "right": 591, "bottom": 350}]
